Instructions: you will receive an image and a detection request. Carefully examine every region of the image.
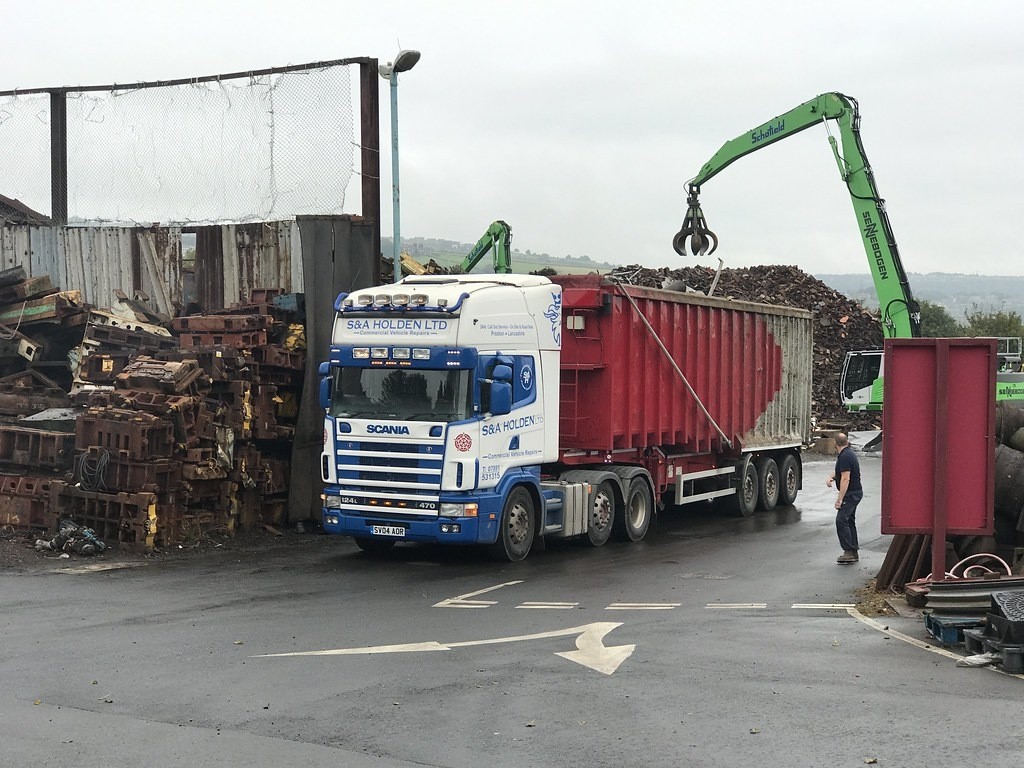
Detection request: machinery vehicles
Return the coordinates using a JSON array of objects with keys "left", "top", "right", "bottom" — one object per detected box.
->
[
  {"left": 673, "top": 89, "right": 1024, "bottom": 413},
  {"left": 453, "top": 220, "right": 514, "bottom": 274}
]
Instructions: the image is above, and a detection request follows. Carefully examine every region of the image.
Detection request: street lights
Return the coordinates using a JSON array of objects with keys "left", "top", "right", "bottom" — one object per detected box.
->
[{"left": 377, "top": 49, "right": 421, "bottom": 282}]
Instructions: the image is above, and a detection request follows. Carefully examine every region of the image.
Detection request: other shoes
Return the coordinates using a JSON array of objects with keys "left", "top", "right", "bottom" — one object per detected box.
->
[{"left": 838, "top": 549, "right": 859, "bottom": 563}]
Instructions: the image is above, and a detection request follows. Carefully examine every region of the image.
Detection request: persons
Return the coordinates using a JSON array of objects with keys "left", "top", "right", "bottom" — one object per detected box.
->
[{"left": 826, "top": 432, "right": 864, "bottom": 562}]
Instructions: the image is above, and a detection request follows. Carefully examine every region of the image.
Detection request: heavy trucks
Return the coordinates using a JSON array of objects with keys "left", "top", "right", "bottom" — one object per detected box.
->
[{"left": 318, "top": 270, "right": 816, "bottom": 563}]
